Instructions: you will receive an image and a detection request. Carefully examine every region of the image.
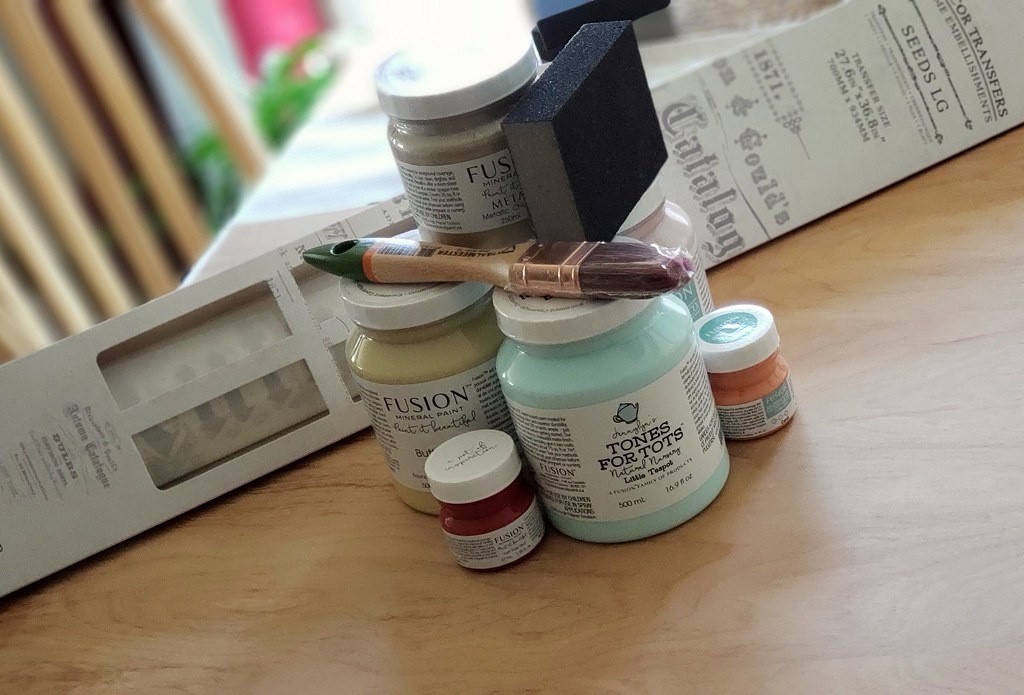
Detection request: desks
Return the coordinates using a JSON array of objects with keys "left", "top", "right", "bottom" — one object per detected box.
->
[{"left": 0, "top": 0, "right": 1024, "bottom": 695}]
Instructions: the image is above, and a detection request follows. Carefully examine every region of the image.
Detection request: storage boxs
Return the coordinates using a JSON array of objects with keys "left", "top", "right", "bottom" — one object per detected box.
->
[{"left": 0, "top": 0, "right": 1024, "bottom": 601}]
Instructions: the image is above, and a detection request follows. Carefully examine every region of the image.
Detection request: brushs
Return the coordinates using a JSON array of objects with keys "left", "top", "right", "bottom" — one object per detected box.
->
[{"left": 300, "top": 238, "right": 688, "bottom": 301}]
